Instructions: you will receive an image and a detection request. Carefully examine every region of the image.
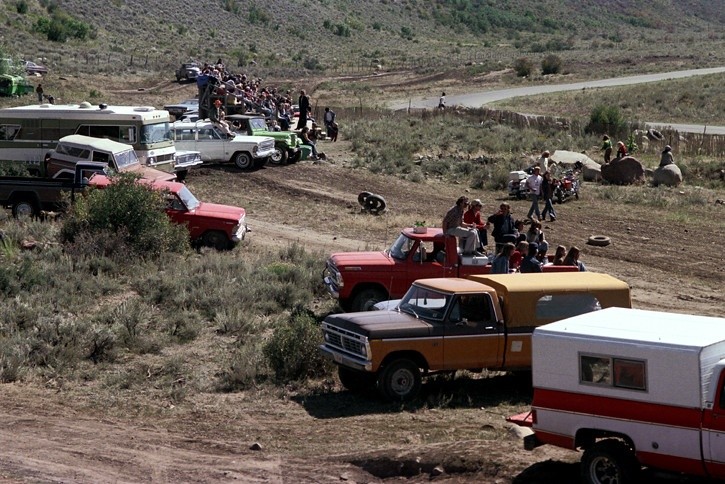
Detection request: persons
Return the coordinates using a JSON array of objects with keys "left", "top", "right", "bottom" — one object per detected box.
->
[
  {"left": 617, "top": 141, "right": 627, "bottom": 158},
  {"left": 36, "top": 84, "right": 43, "bottom": 102},
  {"left": 331, "top": 123, "right": 339, "bottom": 142},
  {"left": 659, "top": 145, "right": 674, "bottom": 167},
  {"left": 447, "top": 294, "right": 487, "bottom": 333},
  {"left": 202, "top": 59, "right": 295, "bottom": 128},
  {"left": 300, "top": 123, "right": 318, "bottom": 155},
  {"left": 600, "top": 135, "right": 613, "bottom": 162},
  {"left": 525, "top": 166, "right": 557, "bottom": 221},
  {"left": 295, "top": 90, "right": 309, "bottom": 130},
  {"left": 440, "top": 92, "right": 446, "bottom": 111},
  {"left": 442, "top": 196, "right": 585, "bottom": 275},
  {"left": 537, "top": 151, "right": 550, "bottom": 173},
  {"left": 209, "top": 100, "right": 234, "bottom": 137},
  {"left": 323, "top": 108, "right": 335, "bottom": 137}
]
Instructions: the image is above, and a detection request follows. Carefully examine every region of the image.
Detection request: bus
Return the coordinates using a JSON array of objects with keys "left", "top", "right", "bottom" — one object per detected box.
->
[{"left": 0, "top": 102, "right": 176, "bottom": 177}]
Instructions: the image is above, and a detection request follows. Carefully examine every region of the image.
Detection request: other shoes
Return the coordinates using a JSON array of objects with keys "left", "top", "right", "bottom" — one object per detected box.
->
[
  {"left": 227, "top": 132, "right": 235, "bottom": 138},
  {"left": 527, "top": 214, "right": 533, "bottom": 220},
  {"left": 313, "top": 155, "right": 319, "bottom": 160}
]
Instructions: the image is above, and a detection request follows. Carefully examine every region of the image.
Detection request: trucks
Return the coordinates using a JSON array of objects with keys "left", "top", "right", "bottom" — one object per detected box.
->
[{"left": 505, "top": 306, "right": 725, "bottom": 484}]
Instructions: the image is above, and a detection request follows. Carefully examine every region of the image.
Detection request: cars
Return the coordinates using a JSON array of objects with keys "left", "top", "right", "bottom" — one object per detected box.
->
[{"left": 373, "top": 298, "right": 446, "bottom": 311}]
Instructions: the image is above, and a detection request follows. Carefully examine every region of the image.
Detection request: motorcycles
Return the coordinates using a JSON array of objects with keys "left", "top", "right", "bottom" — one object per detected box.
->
[{"left": 507, "top": 161, "right": 583, "bottom": 204}]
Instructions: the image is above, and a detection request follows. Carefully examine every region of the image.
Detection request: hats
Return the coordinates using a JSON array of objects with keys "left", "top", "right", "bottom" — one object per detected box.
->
[
  {"left": 471, "top": 199, "right": 485, "bottom": 206},
  {"left": 528, "top": 242, "right": 539, "bottom": 250}
]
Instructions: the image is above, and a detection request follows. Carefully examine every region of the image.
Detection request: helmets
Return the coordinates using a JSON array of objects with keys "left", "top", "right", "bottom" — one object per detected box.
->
[{"left": 213, "top": 100, "right": 222, "bottom": 105}]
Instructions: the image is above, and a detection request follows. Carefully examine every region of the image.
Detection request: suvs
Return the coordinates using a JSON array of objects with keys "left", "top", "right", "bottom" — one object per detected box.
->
[
  {"left": 1, "top": 58, "right": 47, "bottom": 76},
  {"left": 85, "top": 172, "right": 248, "bottom": 251},
  {"left": 44, "top": 134, "right": 177, "bottom": 183},
  {"left": 150, "top": 98, "right": 313, "bottom": 171}
]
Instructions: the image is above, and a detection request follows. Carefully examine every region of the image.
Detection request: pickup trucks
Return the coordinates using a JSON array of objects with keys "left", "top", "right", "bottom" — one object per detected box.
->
[
  {"left": 0, "top": 161, "right": 116, "bottom": 222},
  {"left": 321, "top": 228, "right": 588, "bottom": 312},
  {"left": 318, "top": 271, "right": 632, "bottom": 404},
  {"left": 175, "top": 63, "right": 201, "bottom": 81}
]
